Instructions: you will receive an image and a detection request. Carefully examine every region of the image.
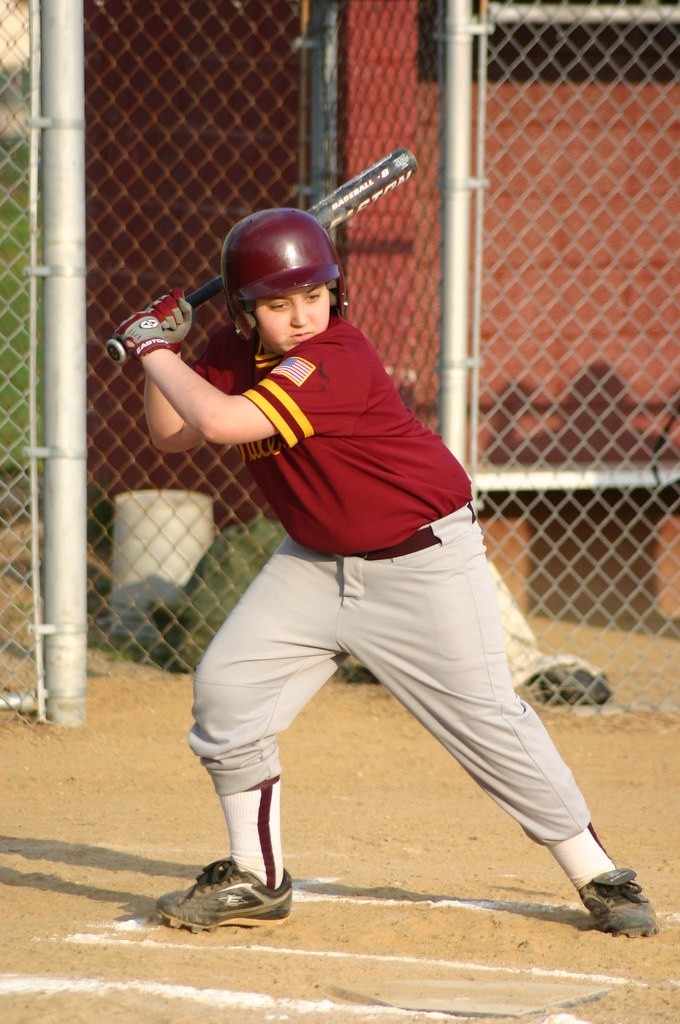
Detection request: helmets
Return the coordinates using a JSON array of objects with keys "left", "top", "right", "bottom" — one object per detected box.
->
[{"left": 221, "top": 206, "right": 347, "bottom": 340}]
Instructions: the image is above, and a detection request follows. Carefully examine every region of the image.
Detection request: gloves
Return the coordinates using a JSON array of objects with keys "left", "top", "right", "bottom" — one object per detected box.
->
[
  {"left": 141, "top": 287, "right": 193, "bottom": 355},
  {"left": 114, "top": 309, "right": 172, "bottom": 358}
]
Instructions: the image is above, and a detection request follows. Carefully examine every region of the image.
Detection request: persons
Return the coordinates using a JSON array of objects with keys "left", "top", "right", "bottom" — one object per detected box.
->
[{"left": 114, "top": 208, "right": 659, "bottom": 938}]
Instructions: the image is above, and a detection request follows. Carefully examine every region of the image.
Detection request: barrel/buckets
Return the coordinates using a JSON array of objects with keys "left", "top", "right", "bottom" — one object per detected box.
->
[{"left": 109, "top": 490, "right": 214, "bottom": 637}]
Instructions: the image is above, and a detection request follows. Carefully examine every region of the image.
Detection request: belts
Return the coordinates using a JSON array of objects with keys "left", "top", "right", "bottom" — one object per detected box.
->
[{"left": 351, "top": 500, "right": 477, "bottom": 562}]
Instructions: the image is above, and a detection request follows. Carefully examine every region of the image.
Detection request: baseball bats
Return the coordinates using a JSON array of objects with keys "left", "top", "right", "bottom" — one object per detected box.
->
[{"left": 105, "top": 147, "right": 418, "bottom": 365}]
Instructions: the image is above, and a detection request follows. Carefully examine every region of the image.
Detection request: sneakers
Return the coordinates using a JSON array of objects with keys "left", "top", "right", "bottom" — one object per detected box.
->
[
  {"left": 152, "top": 855, "right": 293, "bottom": 932},
  {"left": 576, "top": 867, "right": 661, "bottom": 940}
]
